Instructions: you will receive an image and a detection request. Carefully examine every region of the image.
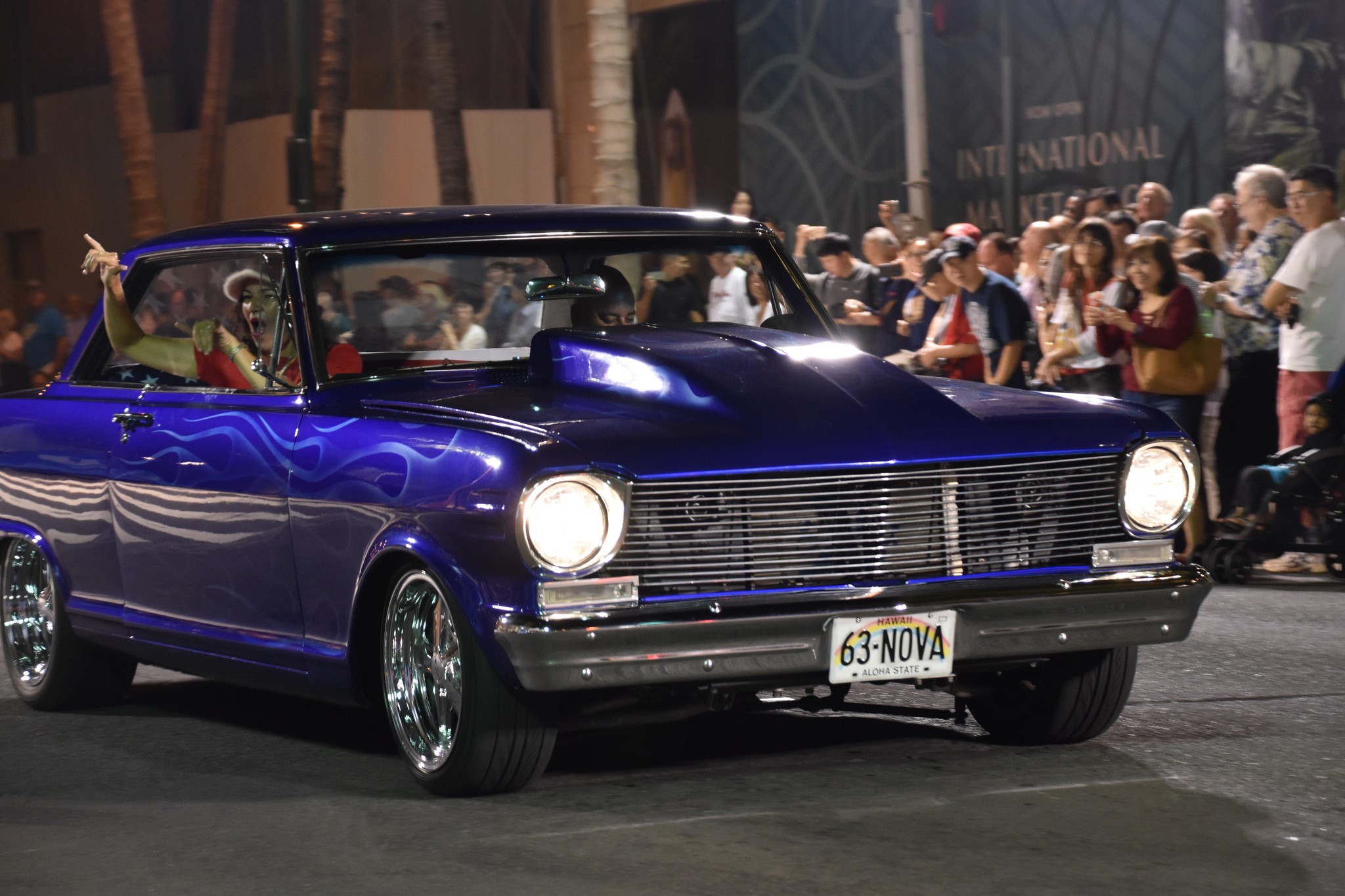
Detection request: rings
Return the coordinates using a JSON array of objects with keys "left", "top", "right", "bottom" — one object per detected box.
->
[{"left": 207, "top": 326, "right": 211, "bottom": 329}]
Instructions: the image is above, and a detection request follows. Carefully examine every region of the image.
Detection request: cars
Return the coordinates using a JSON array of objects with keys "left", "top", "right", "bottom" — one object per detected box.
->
[{"left": 1, "top": 208, "right": 1215, "bottom": 800}]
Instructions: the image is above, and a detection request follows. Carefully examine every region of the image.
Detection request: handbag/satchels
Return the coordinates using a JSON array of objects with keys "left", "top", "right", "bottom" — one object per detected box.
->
[{"left": 1127, "top": 292, "right": 1221, "bottom": 396}]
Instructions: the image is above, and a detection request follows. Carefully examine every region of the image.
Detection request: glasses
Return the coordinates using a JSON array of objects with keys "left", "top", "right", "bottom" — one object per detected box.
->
[
  {"left": 1233, "top": 197, "right": 1257, "bottom": 214},
  {"left": 1283, "top": 193, "right": 1324, "bottom": 204}
]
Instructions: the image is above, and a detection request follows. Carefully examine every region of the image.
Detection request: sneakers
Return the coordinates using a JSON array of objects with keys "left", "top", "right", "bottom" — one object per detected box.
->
[{"left": 1264, "top": 550, "right": 1329, "bottom": 574}]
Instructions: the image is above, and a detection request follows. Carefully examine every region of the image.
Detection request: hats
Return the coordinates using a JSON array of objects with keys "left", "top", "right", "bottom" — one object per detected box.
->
[
  {"left": 941, "top": 223, "right": 982, "bottom": 244},
  {"left": 1123, "top": 220, "right": 1183, "bottom": 244},
  {"left": 222, "top": 264, "right": 287, "bottom": 302},
  {"left": 937, "top": 239, "right": 976, "bottom": 263},
  {"left": 919, "top": 250, "right": 948, "bottom": 285}
]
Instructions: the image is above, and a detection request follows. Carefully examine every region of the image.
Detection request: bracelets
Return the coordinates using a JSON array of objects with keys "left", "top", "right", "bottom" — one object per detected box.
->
[
  {"left": 229, "top": 343, "right": 248, "bottom": 362},
  {"left": 1214, "top": 296, "right": 1225, "bottom": 303}
]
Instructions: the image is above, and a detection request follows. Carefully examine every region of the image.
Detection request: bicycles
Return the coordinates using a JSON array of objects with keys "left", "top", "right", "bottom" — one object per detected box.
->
[{"left": 1213, "top": 444, "right": 1344, "bottom": 580}]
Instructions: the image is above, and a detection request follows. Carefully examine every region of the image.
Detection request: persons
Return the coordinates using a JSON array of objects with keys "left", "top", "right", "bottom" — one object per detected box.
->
[
  {"left": 726, "top": 182, "right": 1261, "bottom": 564},
  {"left": 315, "top": 256, "right": 550, "bottom": 349},
  {"left": 570, "top": 250, "right": 748, "bottom": 328},
  {"left": 1197, "top": 164, "right": 1303, "bottom": 557},
  {"left": 0, "top": 288, "right": 200, "bottom": 393},
  {"left": 81, "top": 233, "right": 362, "bottom": 390},
  {"left": 1215, "top": 393, "right": 1337, "bottom": 531},
  {"left": 1261, "top": 163, "right": 1345, "bottom": 573}
]
